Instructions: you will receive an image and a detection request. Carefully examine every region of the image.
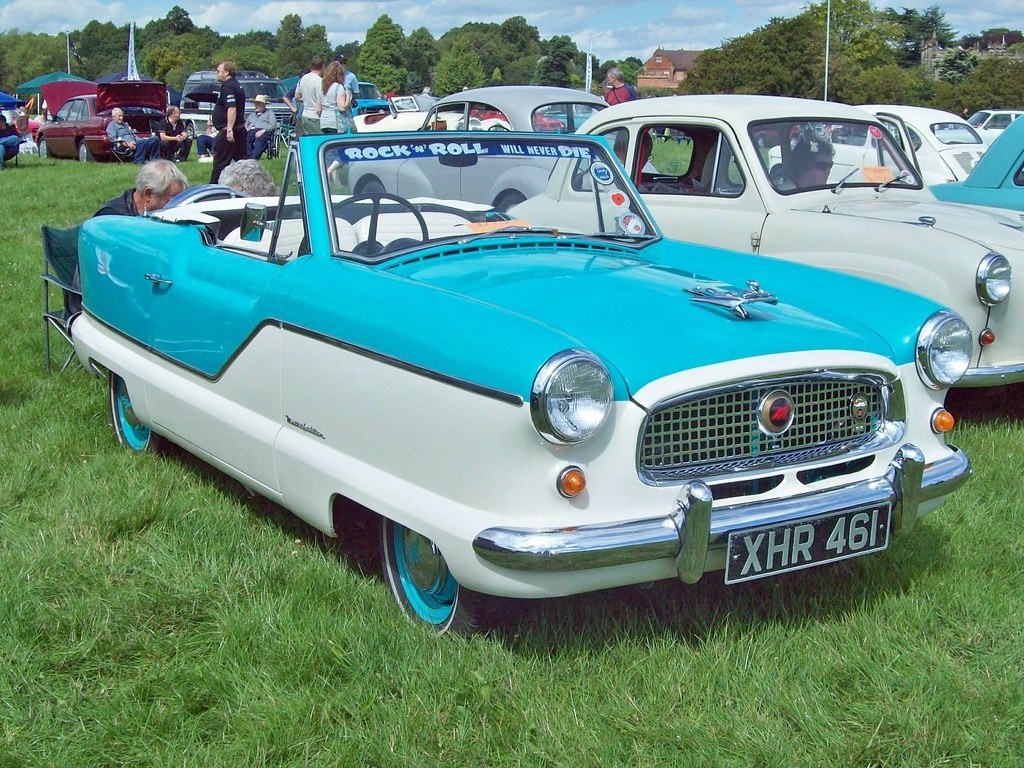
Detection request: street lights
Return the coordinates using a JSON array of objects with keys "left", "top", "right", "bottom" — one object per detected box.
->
[{"left": 585, "top": 32, "right": 605, "bottom": 92}]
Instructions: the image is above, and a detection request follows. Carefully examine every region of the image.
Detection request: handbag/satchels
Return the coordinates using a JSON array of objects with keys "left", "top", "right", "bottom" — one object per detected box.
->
[{"left": 333, "top": 84, "right": 357, "bottom": 134}]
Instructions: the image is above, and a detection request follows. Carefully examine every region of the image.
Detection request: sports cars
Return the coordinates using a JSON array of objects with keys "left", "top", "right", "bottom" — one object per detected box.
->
[{"left": 63, "top": 129, "right": 977, "bottom": 646}]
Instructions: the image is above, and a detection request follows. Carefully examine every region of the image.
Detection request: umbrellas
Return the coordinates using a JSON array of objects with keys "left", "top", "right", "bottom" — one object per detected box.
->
[{"left": 184, "top": 79, "right": 221, "bottom": 123}]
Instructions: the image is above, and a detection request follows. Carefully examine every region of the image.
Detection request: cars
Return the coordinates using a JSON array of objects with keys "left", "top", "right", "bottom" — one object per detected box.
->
[
  {"left": 36, "top": 78, "right": 169, "bottom": 162},
  {"left": 298, "top": 83, "right": 511, "bottom": 131},
  {"left": 11, "top": 114, "right": 41, "bottom": 138},
  {"left": 346, "top": 85, "right": 663, "bottom": 216},
  {"left": 505, "top": 93, "right": 1024, "bottom": 397}
]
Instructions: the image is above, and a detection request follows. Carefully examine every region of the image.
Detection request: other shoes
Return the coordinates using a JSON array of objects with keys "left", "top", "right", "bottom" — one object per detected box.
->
[{"left": 199, "top": 157, "right": 214, "bottom": 162}]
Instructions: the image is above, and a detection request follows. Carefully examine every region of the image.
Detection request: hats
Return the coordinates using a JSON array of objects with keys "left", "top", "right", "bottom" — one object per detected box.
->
[
  {"left": 249, "top": 95, "right": 271, "bottom": 104},
  {"left": 334, "top": 54, "right": 348, "bottom": 62}
]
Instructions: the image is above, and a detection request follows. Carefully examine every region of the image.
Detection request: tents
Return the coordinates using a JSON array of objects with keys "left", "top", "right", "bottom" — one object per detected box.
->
[
  {"left": 14, "top": 71, "right": 88, "bottom": 115},
  {"left": 0, "top": 91, "right": 26, "bottom": 111}
]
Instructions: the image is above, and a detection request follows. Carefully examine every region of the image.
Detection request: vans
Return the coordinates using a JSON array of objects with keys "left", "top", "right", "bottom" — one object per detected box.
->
[{"left": 179, "top": 70, "right": 294, "bottom": 145}]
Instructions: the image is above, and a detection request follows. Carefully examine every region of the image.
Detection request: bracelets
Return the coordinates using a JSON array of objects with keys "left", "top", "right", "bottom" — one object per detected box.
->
[{"left": 228, "top": 130, "right": 231, "bottom": 133}]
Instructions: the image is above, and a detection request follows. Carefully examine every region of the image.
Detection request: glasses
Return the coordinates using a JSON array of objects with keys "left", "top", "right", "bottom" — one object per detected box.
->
[
  {"left": 812, "top": 161, "right": 834, "bottom": 170},
  {"left": 341, "top": 62, "right": 346, "bottom": 64},
  {"left": 646, "top": 152, "right": 654, "bottom": 161}
]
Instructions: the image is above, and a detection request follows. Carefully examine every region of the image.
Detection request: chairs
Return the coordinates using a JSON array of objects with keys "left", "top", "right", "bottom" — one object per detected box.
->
[
  {"left": 4, "top": 123, "right": 27, "bottom": 168},
  {"left": 149, "top": 119, "right": 176, "bottom": 159},
  {"left": 222, "top": 212, "right": 476, "bottom": 260},
  {"left": 280, "top": 114, "right": 296, "bottom": 148},
  {"left": 39, "top": 225, "right": 80, "bottom": 377},
  {"left": 109, "top": 140, "right": 135, "bottom": 164}
]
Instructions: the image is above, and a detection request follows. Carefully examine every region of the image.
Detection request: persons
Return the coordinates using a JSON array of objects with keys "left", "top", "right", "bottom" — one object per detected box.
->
[
  {"left": 217, "top": 159, "right": 277, "bottom": 197},
  {"left": 335, "top": 54, "right": 360, "bottom": 132},
  {"left": 960, "top": 108, "right": 970, "bottom": 120},
  {"left": 317, "top": 61, "right": 351, "bottom": 186},
  {"left": 575, "top": 131, "right": 654, "bottom": 191},
  {"left": 197, "top": 120, "right": 219, "bottom": 162},
  {"left": 776, "top": 133, "right": 836, "bottom": 190},
  {"left": 209, "top": 61, "right": 248, "bottom": 183},
  {"left": 382, "top": 85, "right": 402, "bottom": 98},
  {"left": 68, "top": 160, "right": 189, "bottom": 312},
  {"left": 245, "top": 94, "right": 277, "bottom": 160},
  {"left": 421, "top": 87, "right": 430, "bottom": 96},
  {"left": 106, "top": 107, "right": 159, "bottom": 163},
  {"left": 282, "top": 54, "right": 326, "bottom": 139},
  {"left": 605, "top": 67, "right": 638, "bottom": 105},
  {"left": 0, "top": 106, "right": 45, "bottom": 169},
  {"left": 155, "top": 106, "right": 192, "bottom": 163}
]
